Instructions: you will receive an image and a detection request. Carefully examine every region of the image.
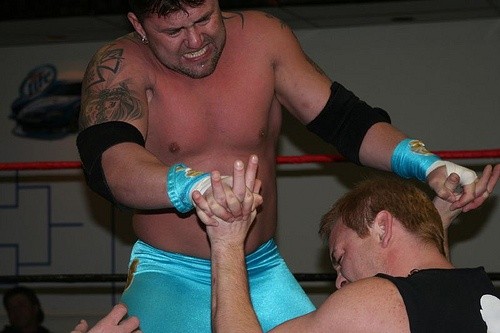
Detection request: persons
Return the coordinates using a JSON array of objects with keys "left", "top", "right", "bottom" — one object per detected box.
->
[
  {"left": 77, "top": 0, "right": 479, "bottom": 333},
  {"left": 193, "top": 154, "right": 499, "bottom": 332},
  {"left": 70, "top": 302, "right": 142, "bottom": 333},
  {"left": 1, "top": 287, "right": 50, "bottom": 333}
]
